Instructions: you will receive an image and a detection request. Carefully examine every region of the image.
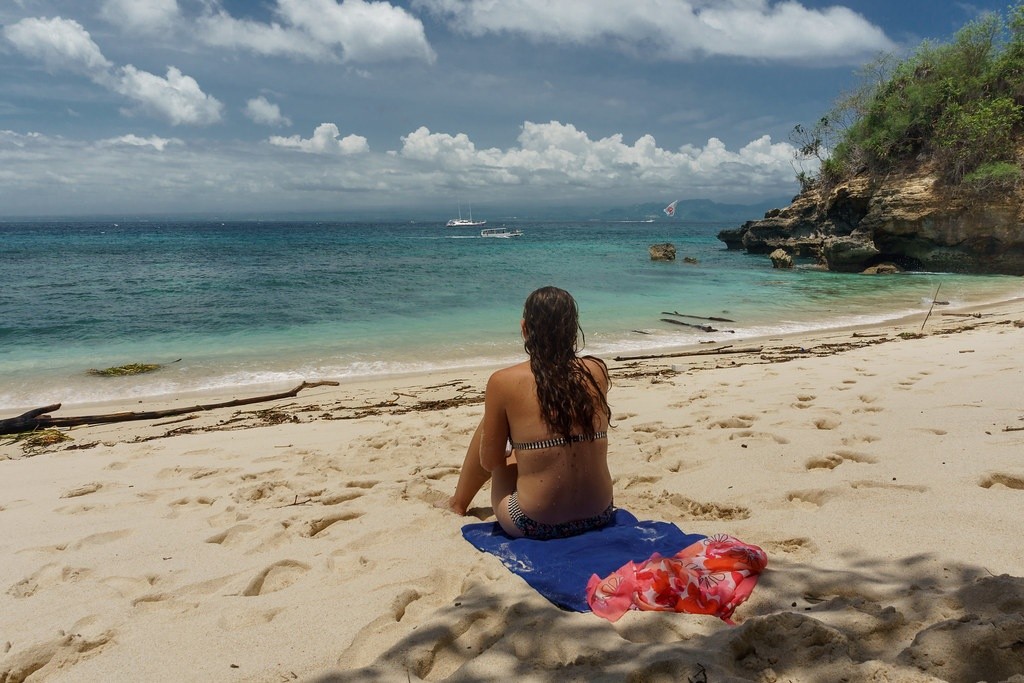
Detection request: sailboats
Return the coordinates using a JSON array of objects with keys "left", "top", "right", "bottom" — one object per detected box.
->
[{"left": 445, "top": 198, "right": 488, "bottom": 227}]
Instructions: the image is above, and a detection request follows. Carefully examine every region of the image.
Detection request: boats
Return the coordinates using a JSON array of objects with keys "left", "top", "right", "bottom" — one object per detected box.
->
[{"left": 480, "top": 227, "right": 524, "bottom": 238}]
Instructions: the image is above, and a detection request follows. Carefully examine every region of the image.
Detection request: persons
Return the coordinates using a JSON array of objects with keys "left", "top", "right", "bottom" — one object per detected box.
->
[{"left": 434, "top": 286, "right": 617, "bottom": 541}]
